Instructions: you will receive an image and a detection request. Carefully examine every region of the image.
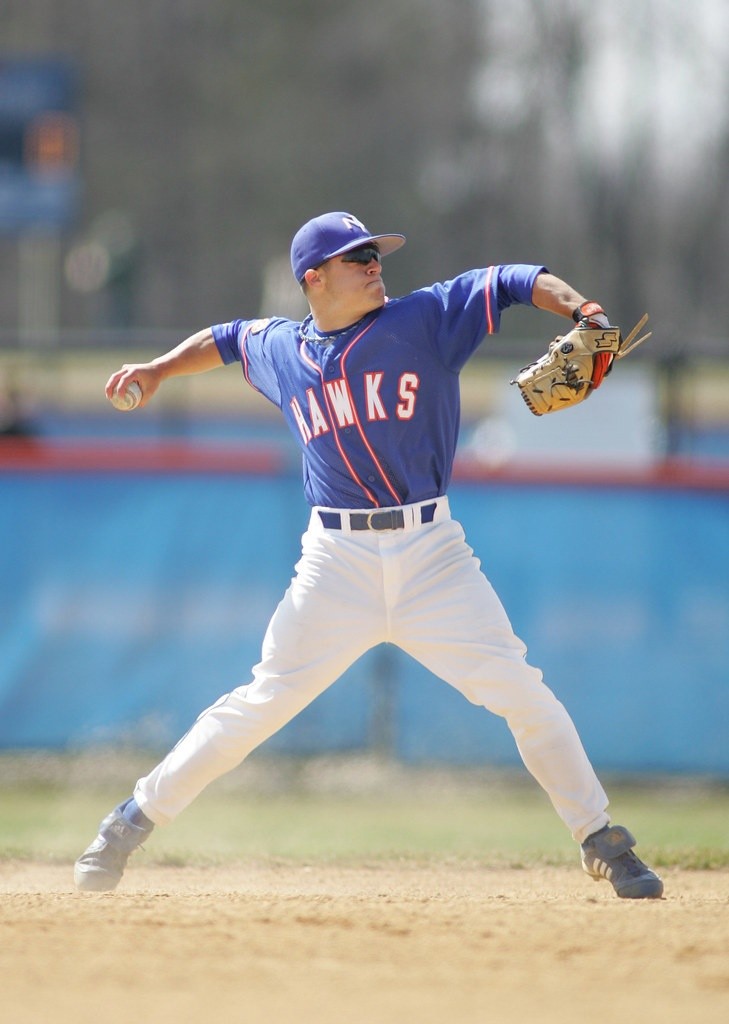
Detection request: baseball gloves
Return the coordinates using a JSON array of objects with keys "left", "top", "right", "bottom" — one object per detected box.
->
[{"left": 508, "top": 311, "right": 624, "bottom": 420}]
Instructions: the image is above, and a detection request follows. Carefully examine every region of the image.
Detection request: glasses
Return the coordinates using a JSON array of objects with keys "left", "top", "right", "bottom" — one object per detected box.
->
[{"left": 314, "top": 243, "right": 382, "bottom": 264}]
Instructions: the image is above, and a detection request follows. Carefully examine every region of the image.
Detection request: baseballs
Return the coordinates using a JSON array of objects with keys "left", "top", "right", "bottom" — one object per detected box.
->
[{"left": 108, "top": 377, "right": 144, "bottom": 414}]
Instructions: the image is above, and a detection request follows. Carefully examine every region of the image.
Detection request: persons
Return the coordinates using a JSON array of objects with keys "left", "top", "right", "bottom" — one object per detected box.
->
[{"left": 73, "top": 212, "right": 664, "bottom": 900}]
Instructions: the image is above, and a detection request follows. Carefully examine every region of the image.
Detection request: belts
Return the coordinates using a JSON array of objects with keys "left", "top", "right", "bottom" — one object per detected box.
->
[{"left": 318, "top": 502, "right": 437, "bottom": 531}]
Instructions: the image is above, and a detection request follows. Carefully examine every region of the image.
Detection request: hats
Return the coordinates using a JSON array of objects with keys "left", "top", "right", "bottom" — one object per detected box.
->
[{"left": 290, "top": 211, "right": 409, "bottom": 284}]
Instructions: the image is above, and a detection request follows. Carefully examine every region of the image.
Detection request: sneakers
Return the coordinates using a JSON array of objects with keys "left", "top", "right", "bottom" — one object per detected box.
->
[
  {"left": 579, "top": 824, "right": 663, "bottom": 898},
  {"left": 75, "top": 796, "right": 154, "bottom": 893}
]
